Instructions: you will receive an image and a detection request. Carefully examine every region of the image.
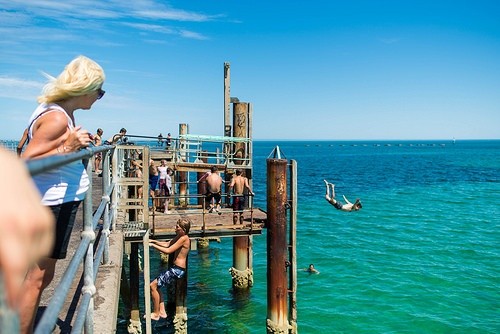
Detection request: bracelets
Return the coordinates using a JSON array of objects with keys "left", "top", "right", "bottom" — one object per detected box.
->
[{"left": 57, "top": 142, "right": 73, "bottom": 154}]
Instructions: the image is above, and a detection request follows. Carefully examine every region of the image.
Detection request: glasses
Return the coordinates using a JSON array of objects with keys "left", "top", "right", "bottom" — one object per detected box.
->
[{"left": 96, "top": 88, "right": 105, "bottom": 99}]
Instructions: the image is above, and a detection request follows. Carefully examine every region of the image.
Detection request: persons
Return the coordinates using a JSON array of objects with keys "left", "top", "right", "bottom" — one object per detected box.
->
[
  {"left": 101, "top": 128, "right": 126, "bottom": 177},
  {"left": 205, "top": 166, "right": 223, "bottom": 215},
  {"left": 0, "top": 143, "right": 56, "bottom": 334},
  {"left": 158, "top": 133, "right": 164, "bottom": 146},
  {"left": 197, "top": 172, "right": 226, "bottom": 213},
  {"left": 17, "top": 127, "right": 30, "bottom": 158},
  {"left": 164, "top": 168, "right": 175, "bottom": 215},
  {"left": 143, "top": 218, "right": 191, "bottom": 321},
  {"left": 157, "top": 160, "right": 169, "bottom": 201},
  {"left": 166, "top": 133, "right": 172, "bottom": 153},
  {"left": 19, "top": 55, "right": 106, "bottom": 334},
  {"left": 126, "top": 148, "right": 159, "bottom": 216},
  {"left": 324, "top": 179, "right": 363, "bottom": 212},
  {"left": 91, "top": 128, "right": 103, "bottom": 174},
  {"left": 297, "top": 264, "right": 320, "bottom": 275},
  {"left": 227, "top": 169, "right": 255, "bottom": 230}
]
